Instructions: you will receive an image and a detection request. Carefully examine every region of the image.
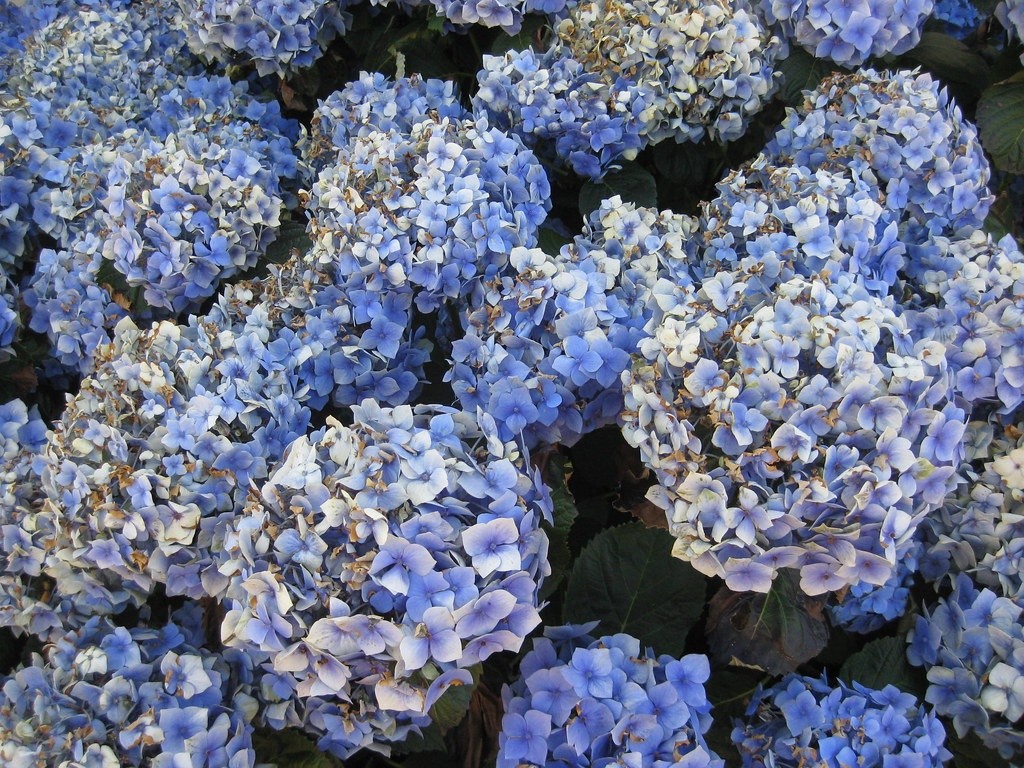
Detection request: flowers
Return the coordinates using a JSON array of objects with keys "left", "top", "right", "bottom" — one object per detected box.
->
[{"left": 0, "top": 0, "right": 1024, "bottom": 768}]
